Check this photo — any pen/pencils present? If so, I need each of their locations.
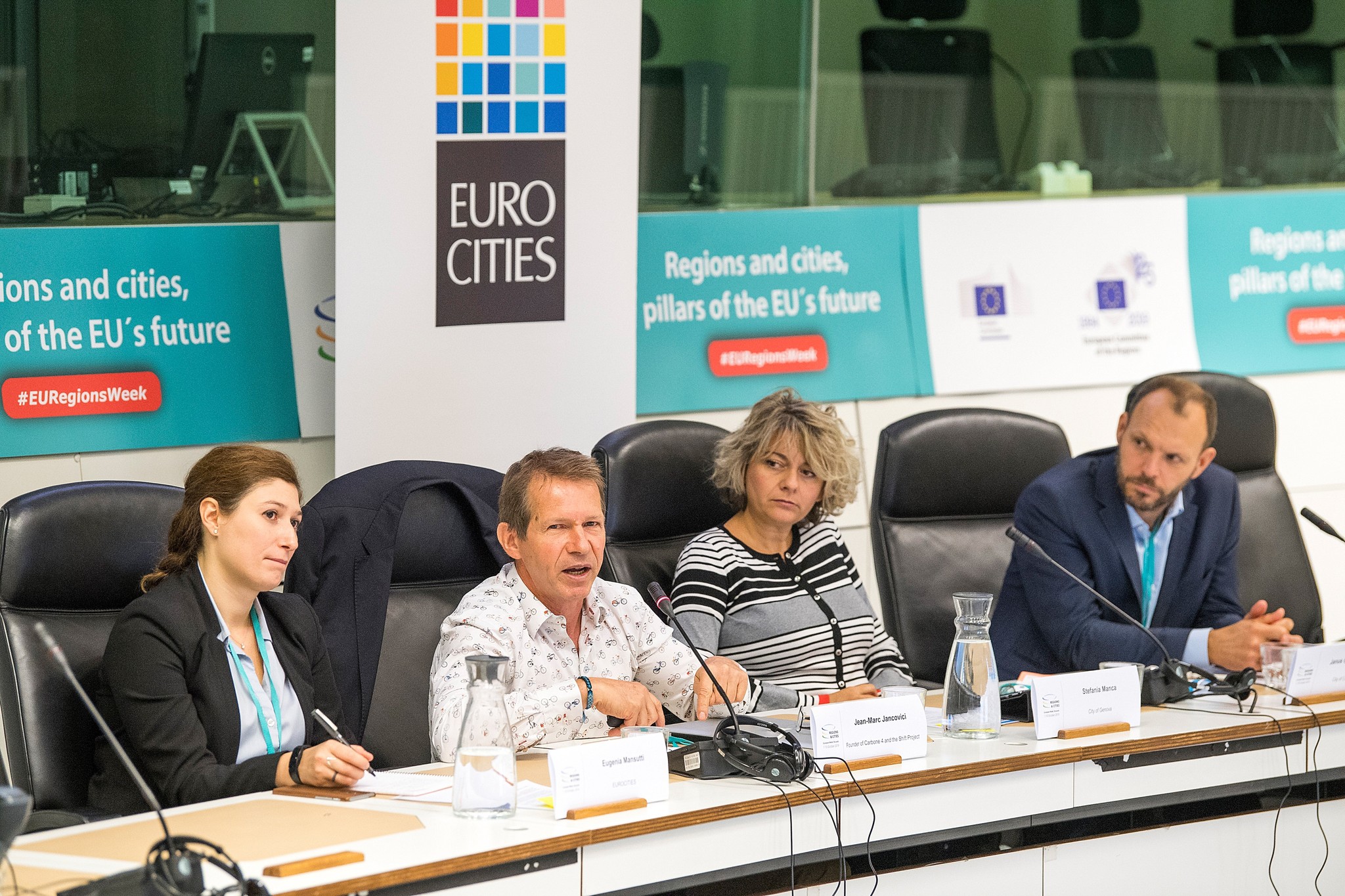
[311,708,377,778]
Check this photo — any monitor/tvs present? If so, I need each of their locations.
[181,28,316,176]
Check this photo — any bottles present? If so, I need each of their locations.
[941,592,1001,740]
[451,655,518,819]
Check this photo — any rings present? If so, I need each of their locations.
[332,771,339,782]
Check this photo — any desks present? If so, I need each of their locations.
[0,663,1345,896]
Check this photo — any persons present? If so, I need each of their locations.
[80,445,374,803]
[667,387,915,707]
[989,374,1308,682]
[425,447,751,766]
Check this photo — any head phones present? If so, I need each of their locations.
[143,834,272,895]
[712,715,816,784]
[1159,655,1257,702]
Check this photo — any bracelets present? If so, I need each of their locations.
[574,674,594,722]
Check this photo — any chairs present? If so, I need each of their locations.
[1214,0,1342,175]
[869,408,1073,689]
[1124,372,1323,645]
[859,0,1006,176]
[282,458,511,772]
[0,476,198,838]
[590,418,746,626]
[639,13,687,198]
[1071,1,1179,178]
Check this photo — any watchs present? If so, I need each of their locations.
[288,745,311,784]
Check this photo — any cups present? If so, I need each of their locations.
[1260,641,1303,690]
[621,725,670,751]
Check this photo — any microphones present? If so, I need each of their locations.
[953,28,1034,191]
[644,581,781,779]
[1001,527,1191,705]
[30,617,182,895]
[1298,508,1345,543]
[1193,35,1272,188]
